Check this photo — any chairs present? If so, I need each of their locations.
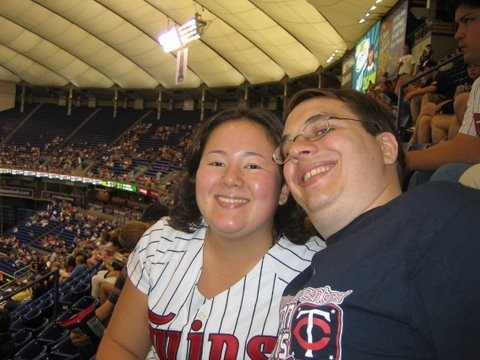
[0,44,480,359]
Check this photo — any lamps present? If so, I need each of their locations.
[156,15,204,54]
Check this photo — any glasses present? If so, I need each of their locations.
[273,118,370,166]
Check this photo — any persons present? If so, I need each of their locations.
[366,0,479,189]
[0,117,198,195]
[1,201,171,347]
[96,107,330,360]
[279,87,480,360]
